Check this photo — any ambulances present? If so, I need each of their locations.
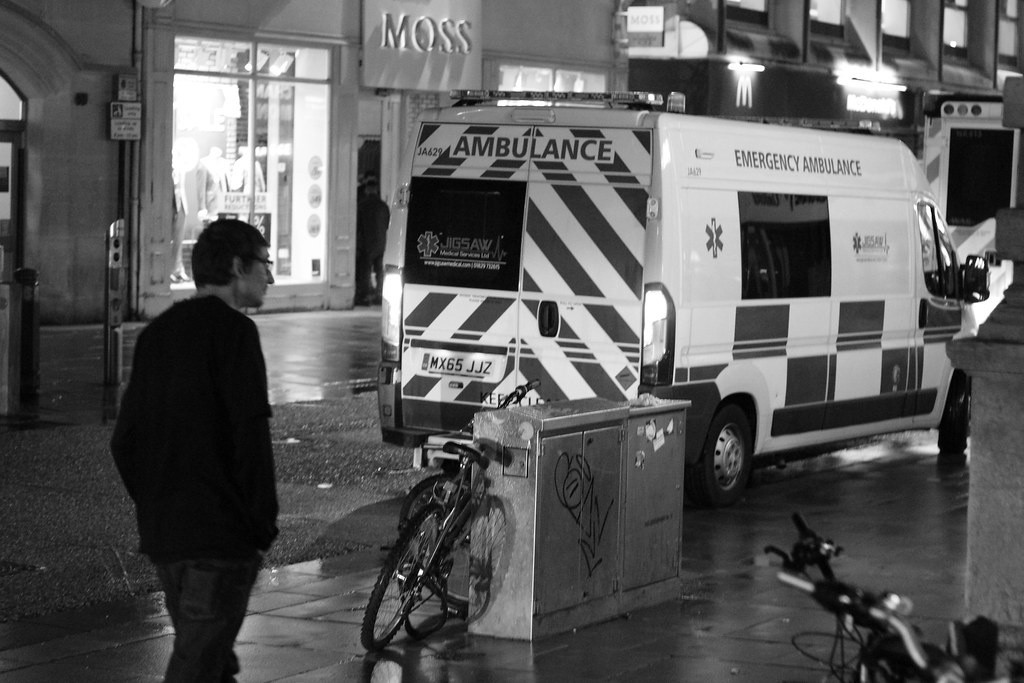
[385,105,990,508]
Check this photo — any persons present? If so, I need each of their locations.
[110,219,282,683]
[358,177,391,306]
[170,145,267,283]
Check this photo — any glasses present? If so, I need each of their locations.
[244,254,273,272]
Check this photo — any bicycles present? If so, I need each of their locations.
[360,377,542,654]
[763,511,1024,683]
